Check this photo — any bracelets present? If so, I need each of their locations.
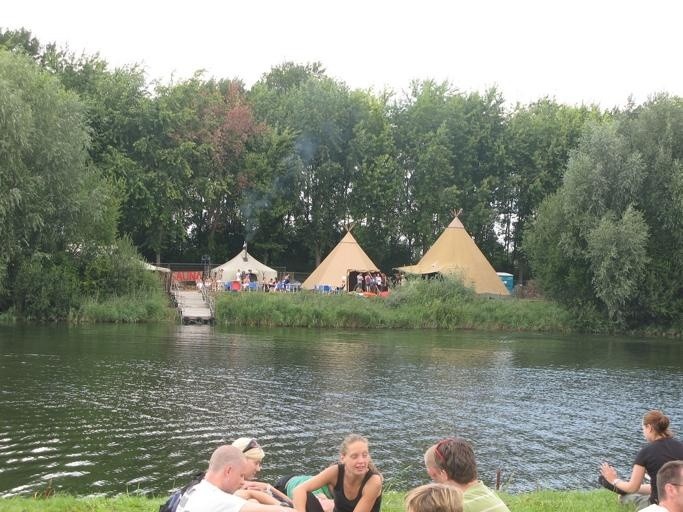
[612,478,620,486]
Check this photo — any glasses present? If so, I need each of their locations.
[435,439,452,463]
[242,440,258,454]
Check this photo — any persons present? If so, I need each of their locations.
[268,273,290,291]
[638,460,683,512]
[424,437,511,512]
[404,483,465,512]
[173,445,302,512]
[236,268,253,283]
[272,472,336,512]
[357,273,409,295]
[195,275,204,290]
[231,437,294,506]
[291,432,383,512]
[597,409,683,508]
